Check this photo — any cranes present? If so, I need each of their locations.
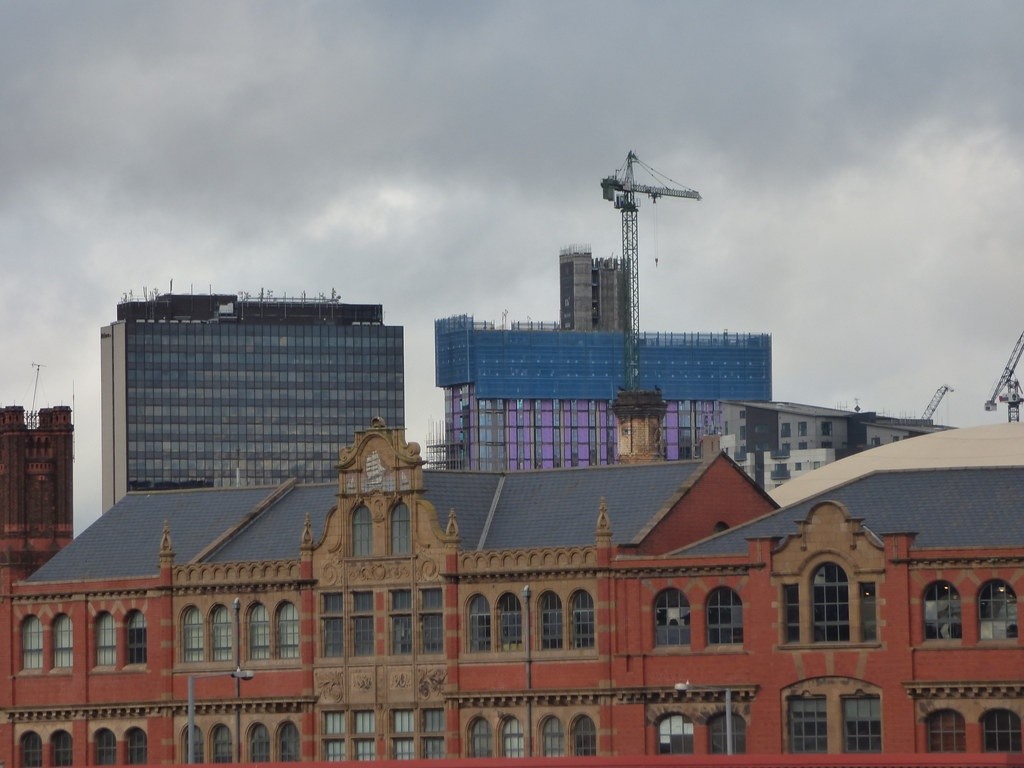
[921,385,957,425]
[986,330,1024,426]
[600,148,702,391]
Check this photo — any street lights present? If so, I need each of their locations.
[675,682,733,756]
[186,669,253,767]
